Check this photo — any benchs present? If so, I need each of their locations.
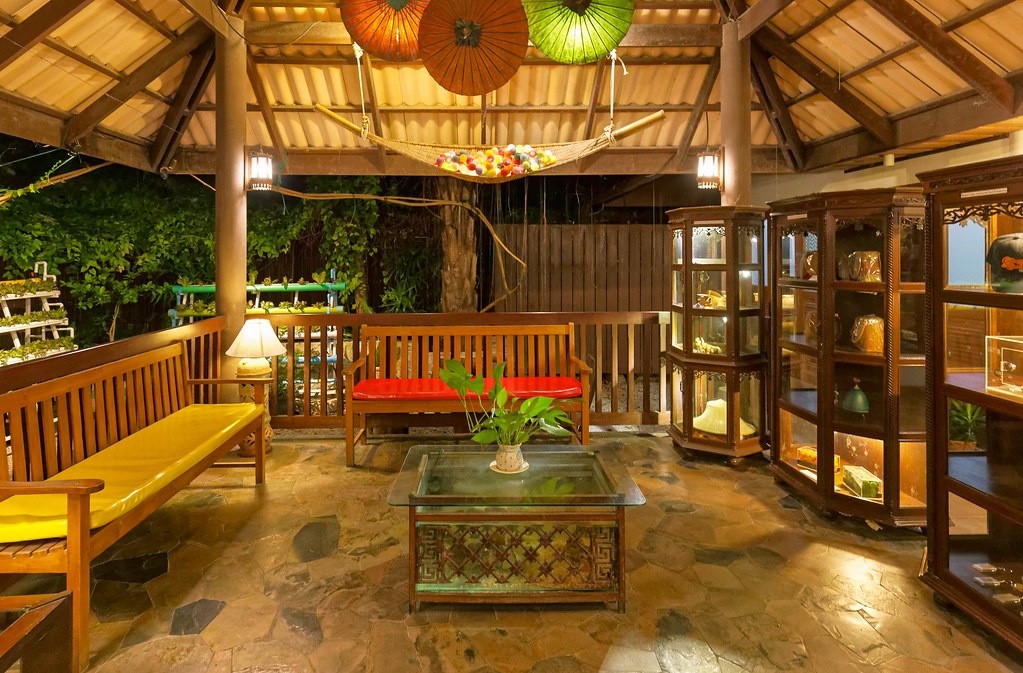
[0,338,276,673]
[341,322,593,465]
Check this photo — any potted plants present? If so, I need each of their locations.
[438,360,585,471]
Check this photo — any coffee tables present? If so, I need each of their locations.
[386,445,648,614]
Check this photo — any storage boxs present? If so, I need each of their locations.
[985,335,1023,398]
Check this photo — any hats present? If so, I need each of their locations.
[986,233,1023,293]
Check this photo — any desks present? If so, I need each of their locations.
[0,589,74,673]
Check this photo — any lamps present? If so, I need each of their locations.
[244,144,273,191]
[225,318,288,458]
[696,145,725,191]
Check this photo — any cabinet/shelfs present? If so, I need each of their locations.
[914,153,1023,652]
[766,185,955,529]
[665,205,770,468]
